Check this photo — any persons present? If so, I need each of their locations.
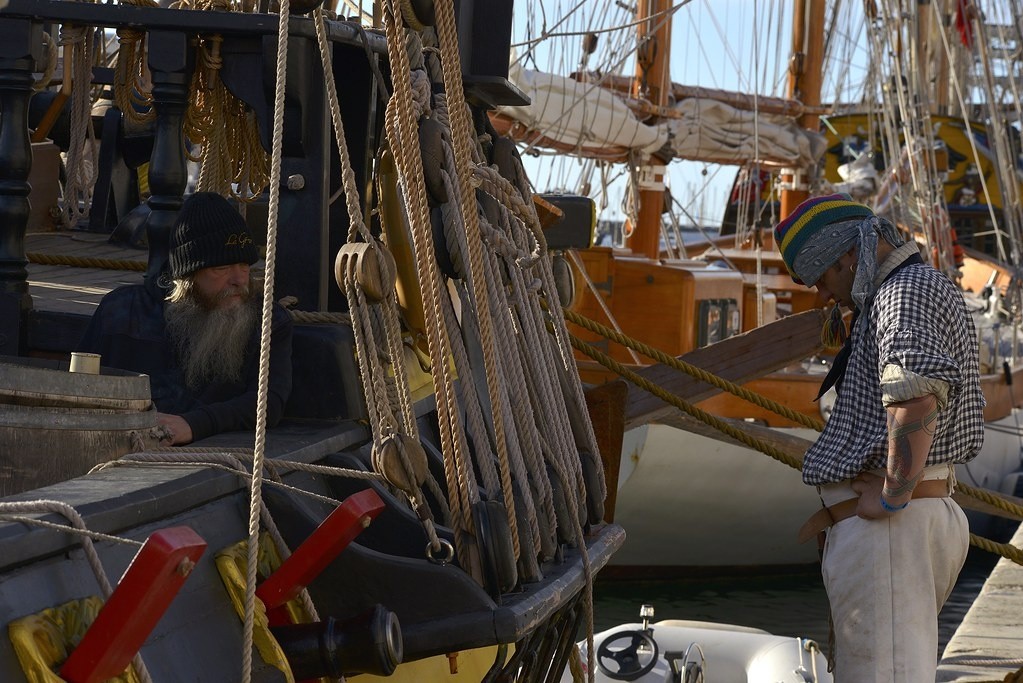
[773,194,985,683]
[77,194,293,446]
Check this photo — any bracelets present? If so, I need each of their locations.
[880,495,911,512]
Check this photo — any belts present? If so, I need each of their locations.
[798,479,949,545]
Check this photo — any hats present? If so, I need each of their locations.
[169,192,260,278]
[774,193,875,282]
